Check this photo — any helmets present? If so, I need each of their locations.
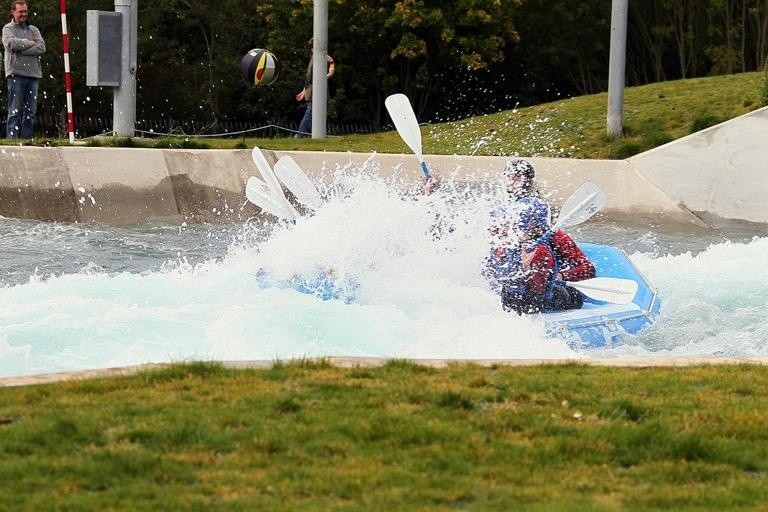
[504,158,536,179]
[510,196,550,228]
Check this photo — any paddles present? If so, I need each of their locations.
[555,278,638,305]
[385,94,437,193]
[245,146,322,225]
[496,181,606,281]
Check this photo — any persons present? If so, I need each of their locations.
[2,0,46,139]
[481,159,596,316]
[418,174,435,196]
[294,37,335,138]
